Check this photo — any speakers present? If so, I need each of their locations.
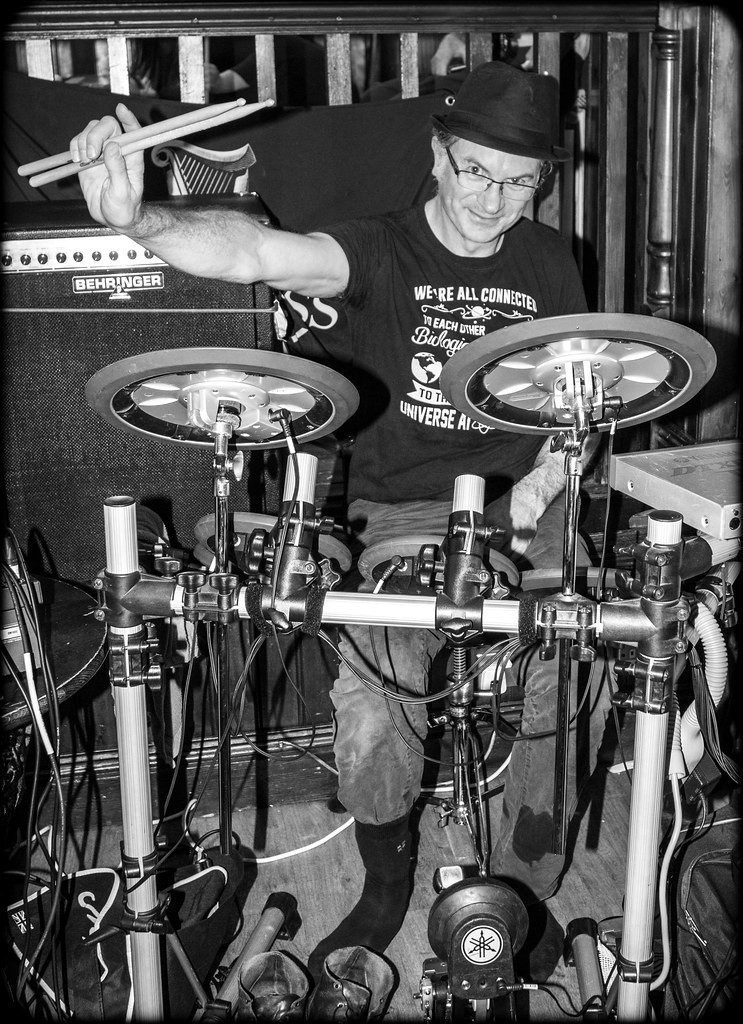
[0,192,302,747]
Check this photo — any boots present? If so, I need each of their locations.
[236,945,396,1024]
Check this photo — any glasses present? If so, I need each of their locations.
[445,144,541,201]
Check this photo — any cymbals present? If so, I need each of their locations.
[435,305,721,440]
[79,341,369,456]
[355,532,523,605]
[520,559,646,605]
[191,506,358,584]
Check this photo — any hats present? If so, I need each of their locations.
[431,60,573,165]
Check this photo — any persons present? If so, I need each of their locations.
[69,60,618,990]
